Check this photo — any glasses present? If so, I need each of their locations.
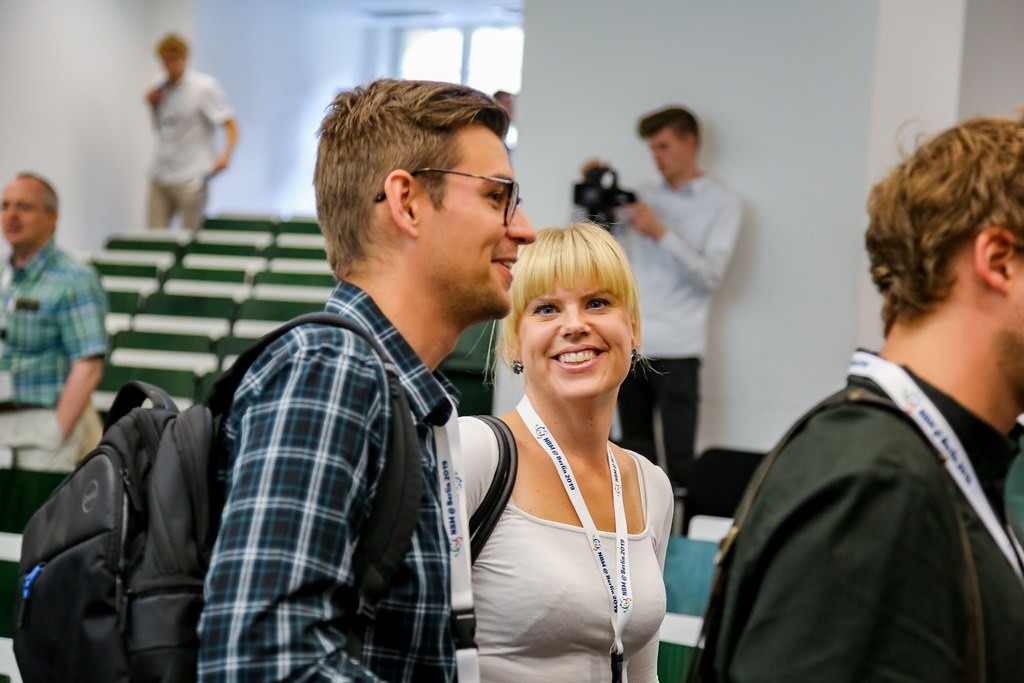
[374,167,521,227]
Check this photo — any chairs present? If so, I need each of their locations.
[1,215,767,683]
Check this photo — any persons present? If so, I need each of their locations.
[198,78,536,683]
[1,172,107,469]
[457,223,674,683]
[583,108,742,482]
[148,34,237,231]
[690,116,1024,683]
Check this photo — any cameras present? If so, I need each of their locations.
[574,167,637,211]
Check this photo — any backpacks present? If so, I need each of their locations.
[13,309,424,683]
